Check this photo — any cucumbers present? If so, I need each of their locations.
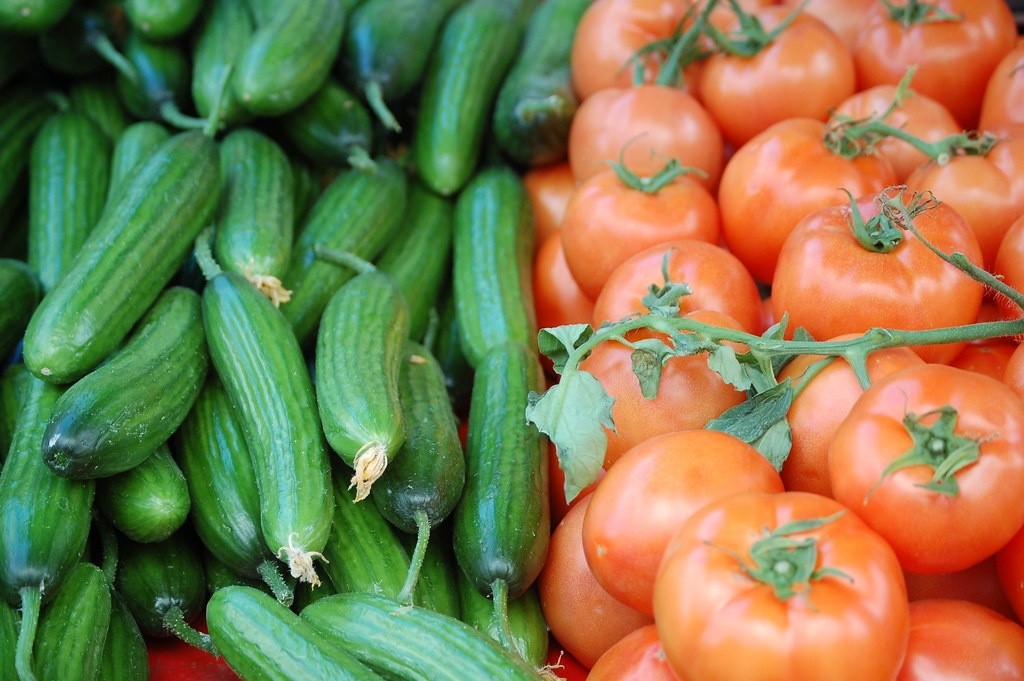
[0,0,595,681]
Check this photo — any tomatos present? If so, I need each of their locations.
[536,0,1024,680]
